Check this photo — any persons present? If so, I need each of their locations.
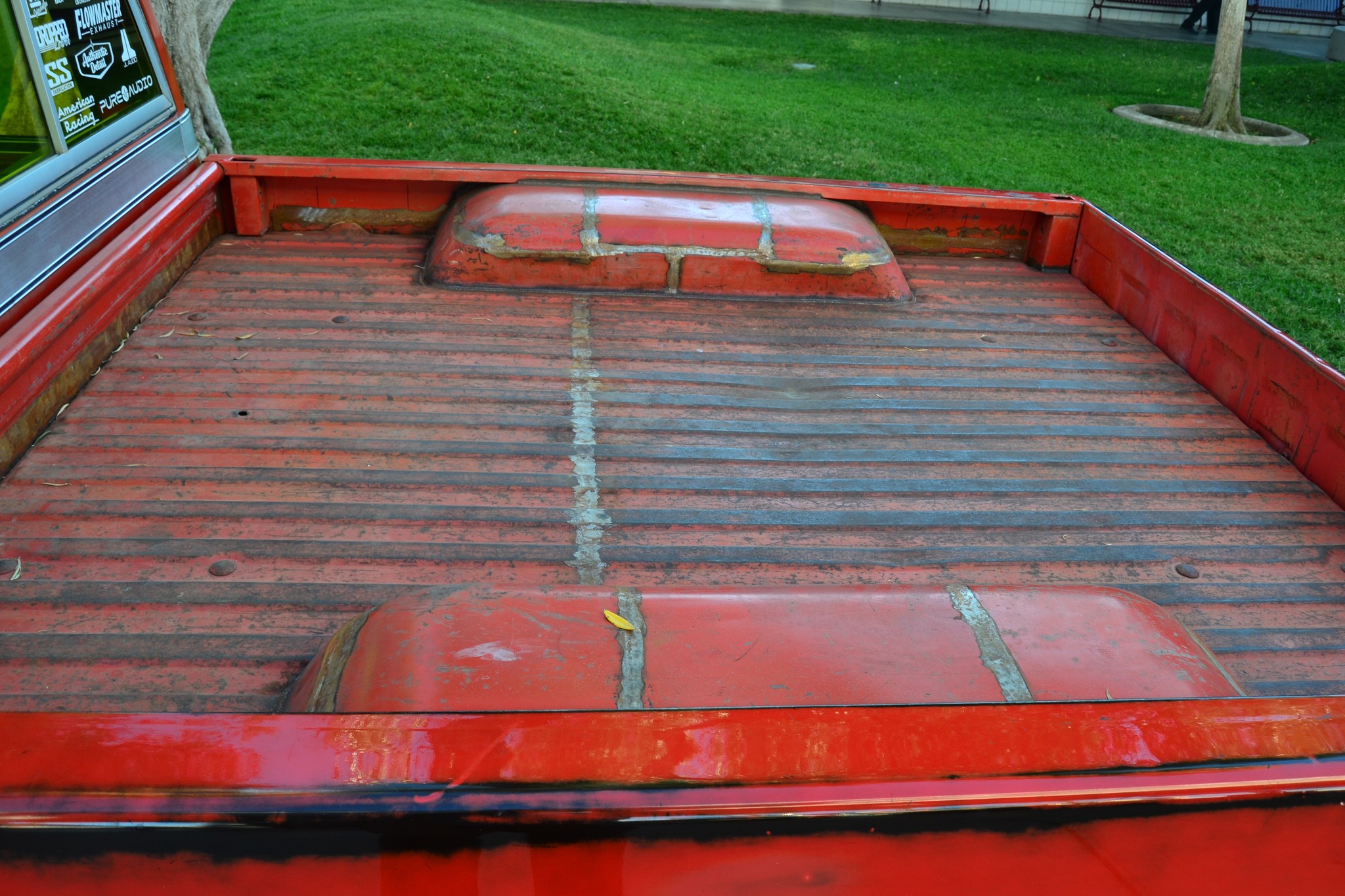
[1181,0,1221,37]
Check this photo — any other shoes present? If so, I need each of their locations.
[1206,30,1219,35]
[1180,19,1198,34]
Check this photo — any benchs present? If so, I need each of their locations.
[1087,0,1203,31]
[1245,0,1345,38]
